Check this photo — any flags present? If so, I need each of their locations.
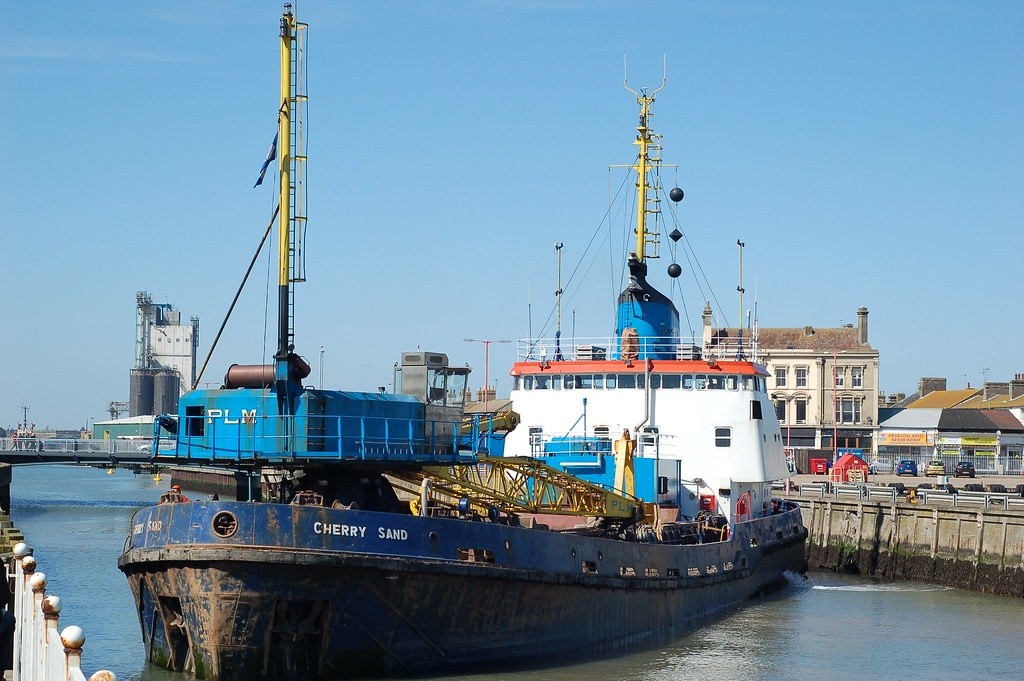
[253,127,278,189]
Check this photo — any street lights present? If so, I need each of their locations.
[319,345,325,391]
[463,338,513,414]
[922,430,927,465]
[86,416,95,433]
[784,395,794,452]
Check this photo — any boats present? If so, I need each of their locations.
[118,54,809,681]
[10,405,38,452]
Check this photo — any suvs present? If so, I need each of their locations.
[953,461,975,478]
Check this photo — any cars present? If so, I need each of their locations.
[924,460,946,477]
[868,459,897,475]
[896,460,917,476]
[137,439,175,454]
